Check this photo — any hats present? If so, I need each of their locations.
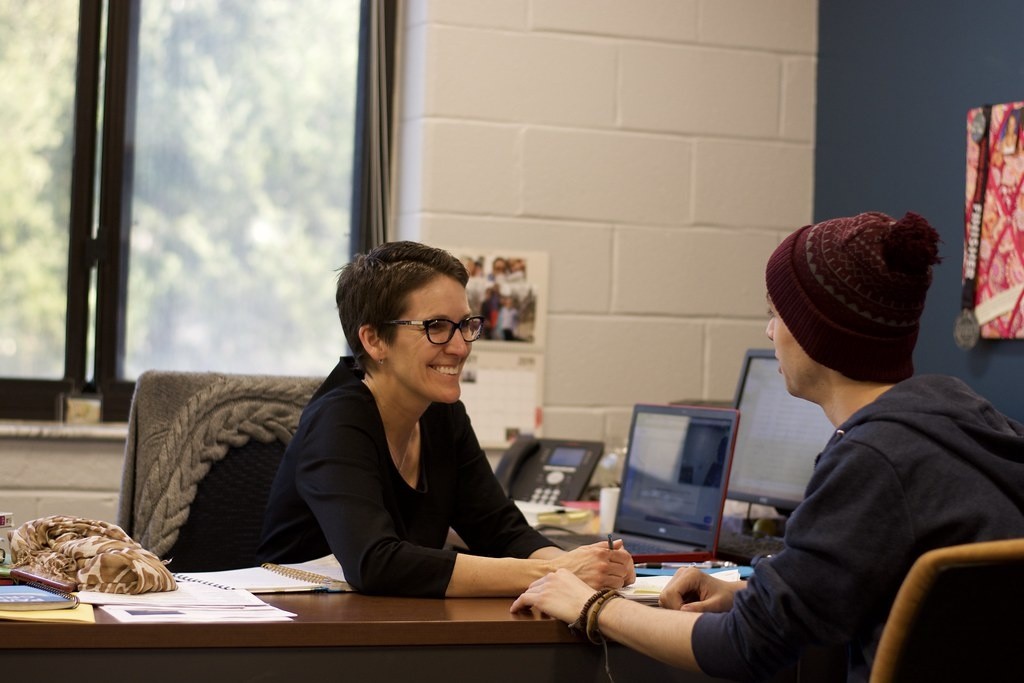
[766,210,946,386]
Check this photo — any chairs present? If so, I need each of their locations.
[115,368,326,573]
[870,538,1024,683]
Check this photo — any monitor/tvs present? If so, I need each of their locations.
[724,348,838,511]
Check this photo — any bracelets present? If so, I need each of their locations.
[568,588,625,645]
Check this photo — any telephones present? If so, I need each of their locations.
[494,435,604,507]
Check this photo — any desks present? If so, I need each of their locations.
[0,587,692,683]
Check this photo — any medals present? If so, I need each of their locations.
[955,311,980,349]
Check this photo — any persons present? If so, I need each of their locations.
[258,241,636,600]
[1001,115,1017,155]
[509,212,1024,683]
[459,254,532,342]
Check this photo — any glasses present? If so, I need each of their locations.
[382,315,485,345]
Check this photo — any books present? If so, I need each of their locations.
[0,581,80,611]
[173,554,358,593]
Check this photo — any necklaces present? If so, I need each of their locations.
[399,426,415,473]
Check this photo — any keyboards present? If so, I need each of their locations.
[716,527,788,564]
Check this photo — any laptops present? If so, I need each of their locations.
[543,403,741,564]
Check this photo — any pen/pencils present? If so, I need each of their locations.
[634,562,722,569]
[608,534,614,550]
